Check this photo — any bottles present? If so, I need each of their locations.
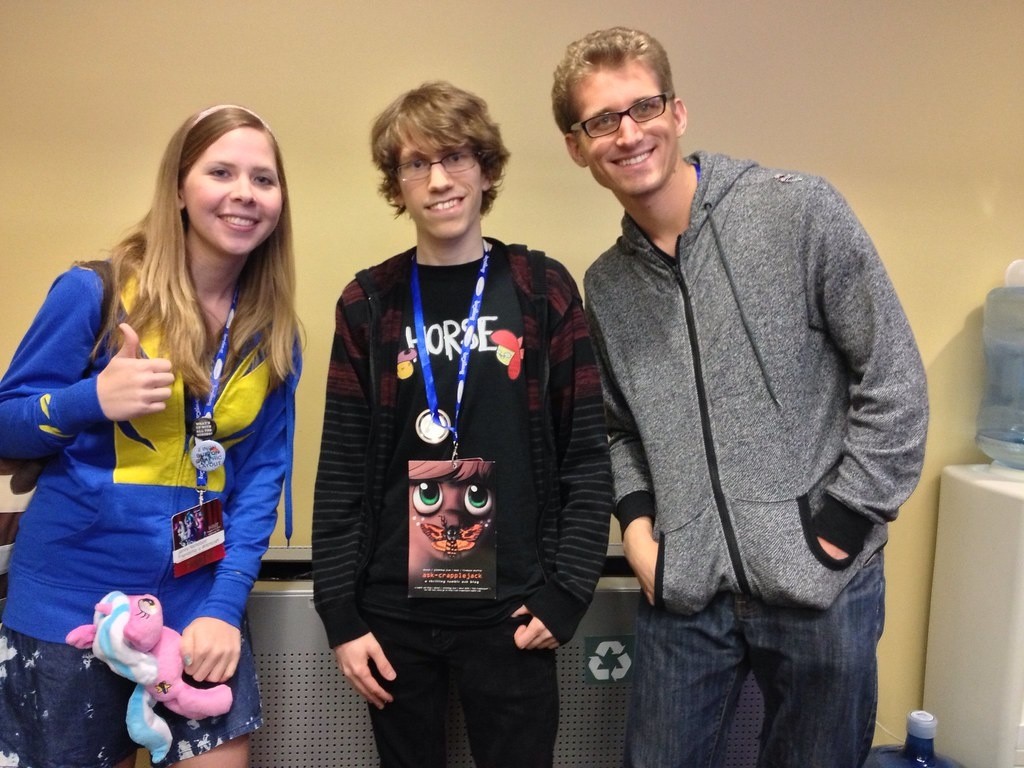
[976,287,1024,470]
[862,710,967,768]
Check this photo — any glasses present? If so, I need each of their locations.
[571,92,670,139]
[388,153,477,181]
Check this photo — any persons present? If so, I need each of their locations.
[306,78,615,768]
[0,102,297,768]
[551,22,931,768]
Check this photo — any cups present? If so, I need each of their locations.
[1005,260,1024,287]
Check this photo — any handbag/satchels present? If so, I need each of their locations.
[0,261,112,599]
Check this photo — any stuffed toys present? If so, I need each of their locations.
[65,590,234,766]
